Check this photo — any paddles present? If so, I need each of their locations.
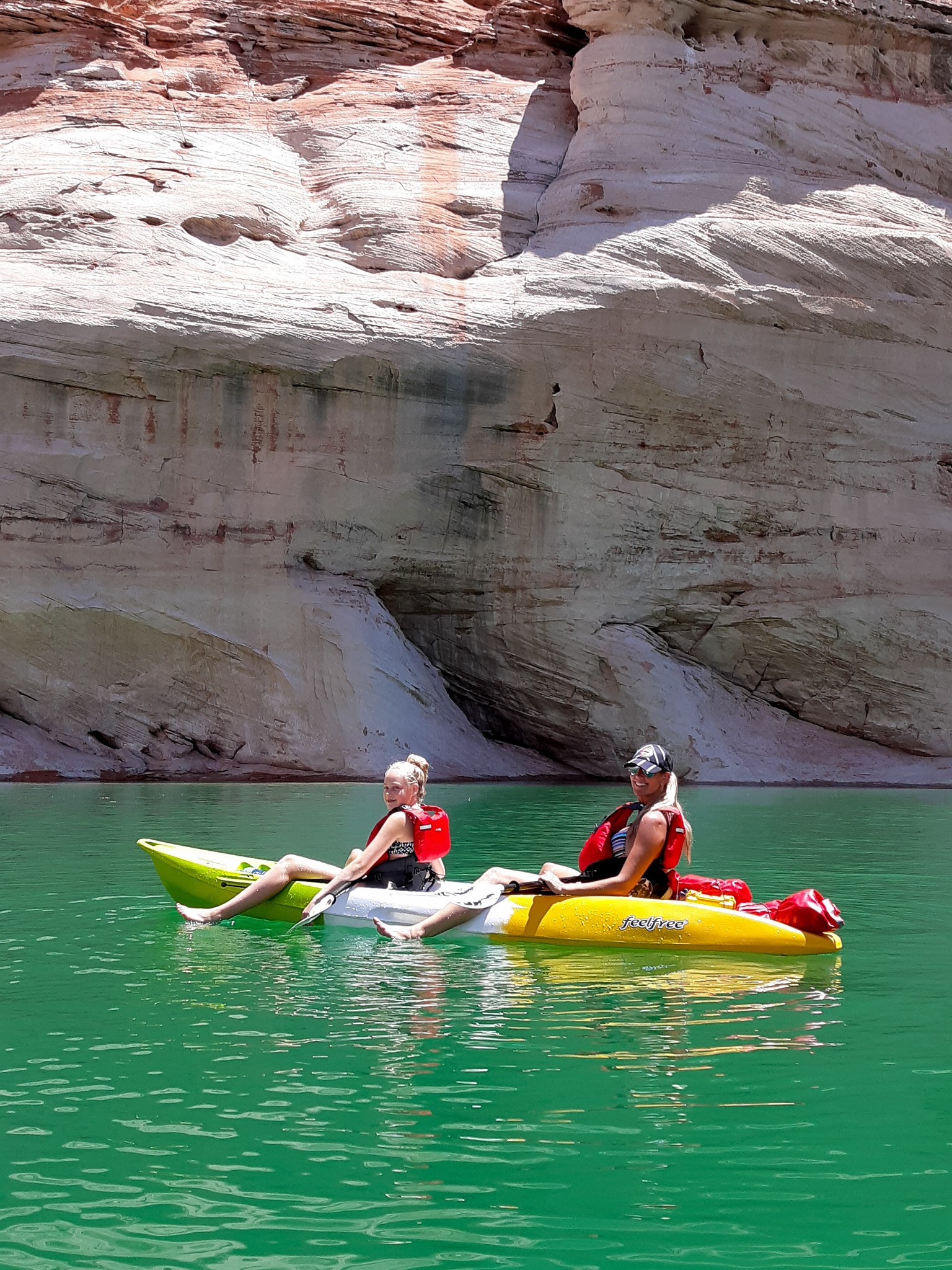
[287,877,363,936]
[443,872,586,911]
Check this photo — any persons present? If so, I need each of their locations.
[176,754,446,926]
[373,745,692,939]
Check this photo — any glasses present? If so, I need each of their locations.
[628,764,666,778]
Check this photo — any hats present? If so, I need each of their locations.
[622,743,675,774]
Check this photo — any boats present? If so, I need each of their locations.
[134,836,846,958]
